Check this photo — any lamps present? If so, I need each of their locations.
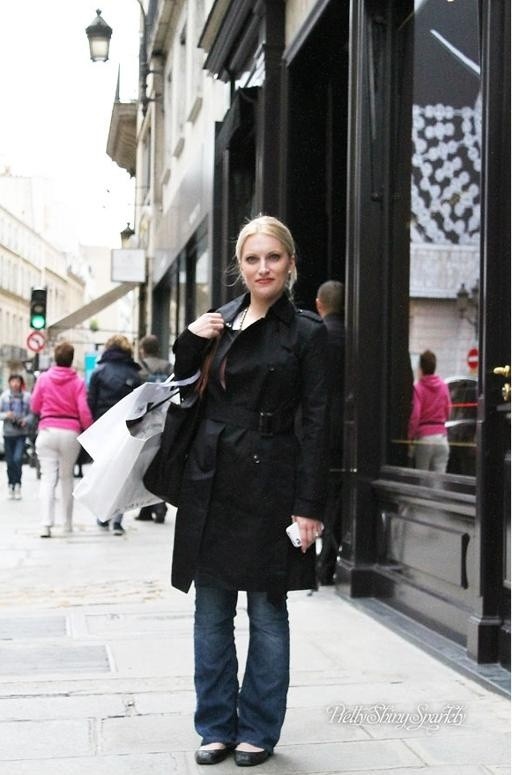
[120,222,134,249]
[85,1,165,116]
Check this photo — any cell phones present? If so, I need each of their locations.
[286,521,324,548]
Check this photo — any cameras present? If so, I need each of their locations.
[10,416,22,429]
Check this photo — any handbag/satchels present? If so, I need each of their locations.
[74,378,198,526]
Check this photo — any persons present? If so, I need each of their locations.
[170,213,334,767]
[314,280,345,588]
[406,349,452,490]
[0,334,174,538]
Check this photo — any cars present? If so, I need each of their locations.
[437,376,478,474]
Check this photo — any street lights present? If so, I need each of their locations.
[457,277,479,342]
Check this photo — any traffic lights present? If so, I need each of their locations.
[28,288,48,331]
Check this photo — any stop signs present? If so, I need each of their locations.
[467,348,479,369]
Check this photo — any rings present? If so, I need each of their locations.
[314,531,321,537]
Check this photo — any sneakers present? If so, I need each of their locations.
[62,522,74,532]
[97,519,124,535]
[39,525,52,538]
[8,481,23,499]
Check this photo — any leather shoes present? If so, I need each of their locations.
[231,743,272,767]
[195,739,236,765]
